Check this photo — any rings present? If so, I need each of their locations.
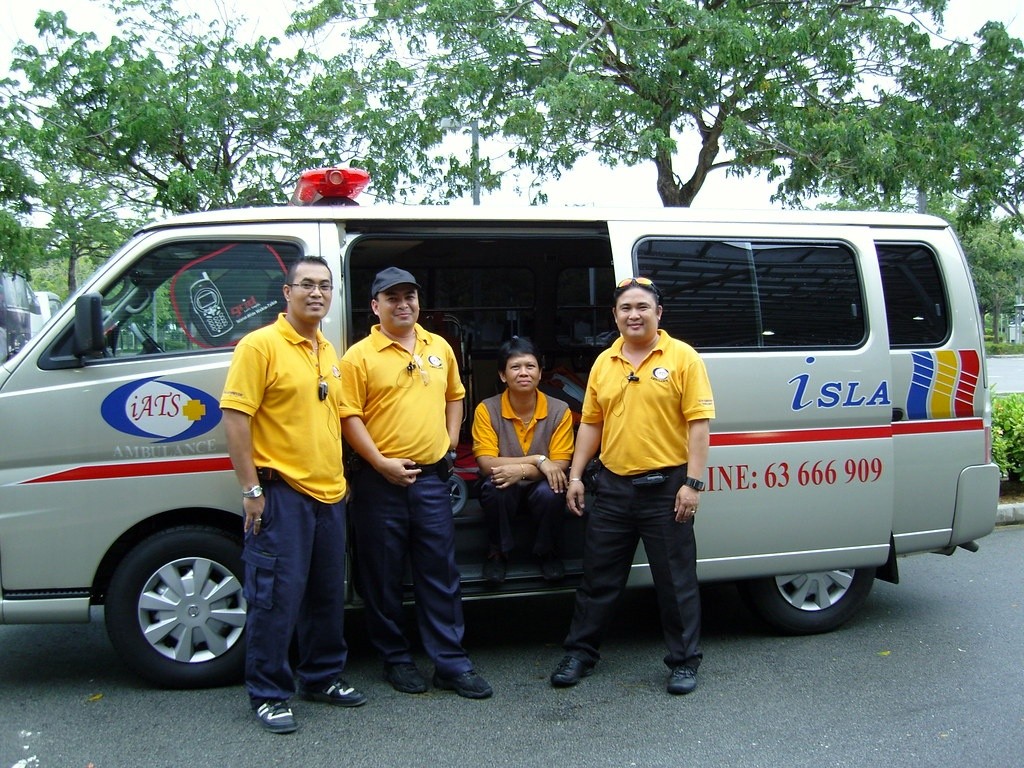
[696,509,698,511]
[691,511,695,514]
[255,518,261,522]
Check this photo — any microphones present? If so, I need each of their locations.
[409,361,413,373]
[629,371,634,383]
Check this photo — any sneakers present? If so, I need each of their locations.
[550,654,593,685]
[382,661,428,693]
[298,675,368,707]
[539,556,566,581]
[432,667,492,699]
[667,664,698,695]
[256,698,298,733]
[481,546,507,582]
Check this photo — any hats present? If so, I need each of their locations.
[372,267,422,299]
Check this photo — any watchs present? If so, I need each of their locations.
[537,455,546,468]
[447,447,458,459]
[684,476,705,491]
[242,485,263,498]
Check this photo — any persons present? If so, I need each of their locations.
[472,337,575,582]
[219,257,368,733]
[551,277,716,693]
[339,267,493,699]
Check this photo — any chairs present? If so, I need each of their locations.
[261,275,288,325]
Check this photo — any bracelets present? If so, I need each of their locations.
[519,463,526,480]
[568,478,580,484]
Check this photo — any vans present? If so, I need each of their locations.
[0,168,1000,690]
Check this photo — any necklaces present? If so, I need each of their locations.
[314,348,319,350]
[512,403,536,424]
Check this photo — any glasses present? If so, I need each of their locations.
[287,281,334,292]
[614,277,660,301]
